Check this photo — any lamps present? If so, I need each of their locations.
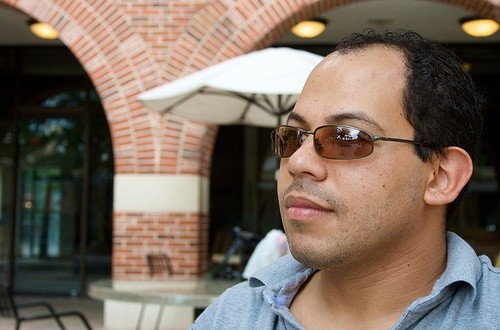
[457,14,500,38]
[290,16,328,39]
[25,18,59,40]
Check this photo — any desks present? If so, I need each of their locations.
[88,275,243,330]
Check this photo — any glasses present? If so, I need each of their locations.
[269,125,443,161]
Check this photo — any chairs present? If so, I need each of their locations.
[0,261,93,330]
[212,239,242,278]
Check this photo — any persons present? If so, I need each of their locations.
[186,26,500,330]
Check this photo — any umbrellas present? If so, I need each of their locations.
[136,45,328,173]
